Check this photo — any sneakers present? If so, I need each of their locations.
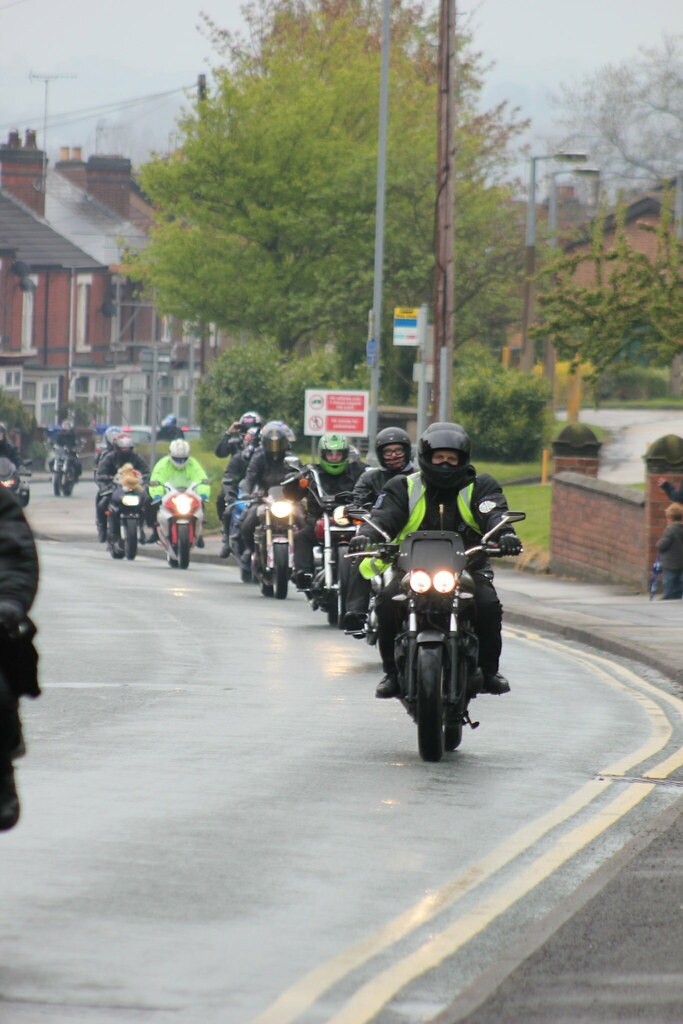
[484,672,511,695]
[375,671,400,699]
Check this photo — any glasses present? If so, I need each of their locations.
[383,448,406,456]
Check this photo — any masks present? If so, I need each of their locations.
[383,456,406,469]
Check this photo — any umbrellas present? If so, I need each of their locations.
[649,552,661,602]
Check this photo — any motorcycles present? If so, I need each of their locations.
[147,478,212,568]
[0,456,33,509]
[346,509,527,761]
[230,491,302,599]
[97,472,150,560]
[337,491,396,645]
[50,443,82,497]
[281,457,372,626]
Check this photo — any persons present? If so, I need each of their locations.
[0,430,41,829]
[216,412,521,700]
[654,476,683,601]
[44,414,212,572]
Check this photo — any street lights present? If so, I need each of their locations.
[519,152,590,365]
[545,167,602,376]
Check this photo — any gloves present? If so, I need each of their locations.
[498,533,522,557]
[349,535,371,557]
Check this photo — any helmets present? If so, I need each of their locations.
[0,423,7,442]
[374,426,411,471]
[61,421,74,436]
[161,415,177,428]
[240,411,295,461]
[105,426,122,446]
[169,439,190,469]
[319,433,349,475]
[113,434,134,455]
[418,422,476,490]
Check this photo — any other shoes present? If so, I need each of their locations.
[291,569,308,590]
[341,610,367,639]
[219,543,230,558]
[147,527,159,543]
[97,526,107,543]
[196,535,205,549]
[0,762,20,832]
[241,550,251,562]
